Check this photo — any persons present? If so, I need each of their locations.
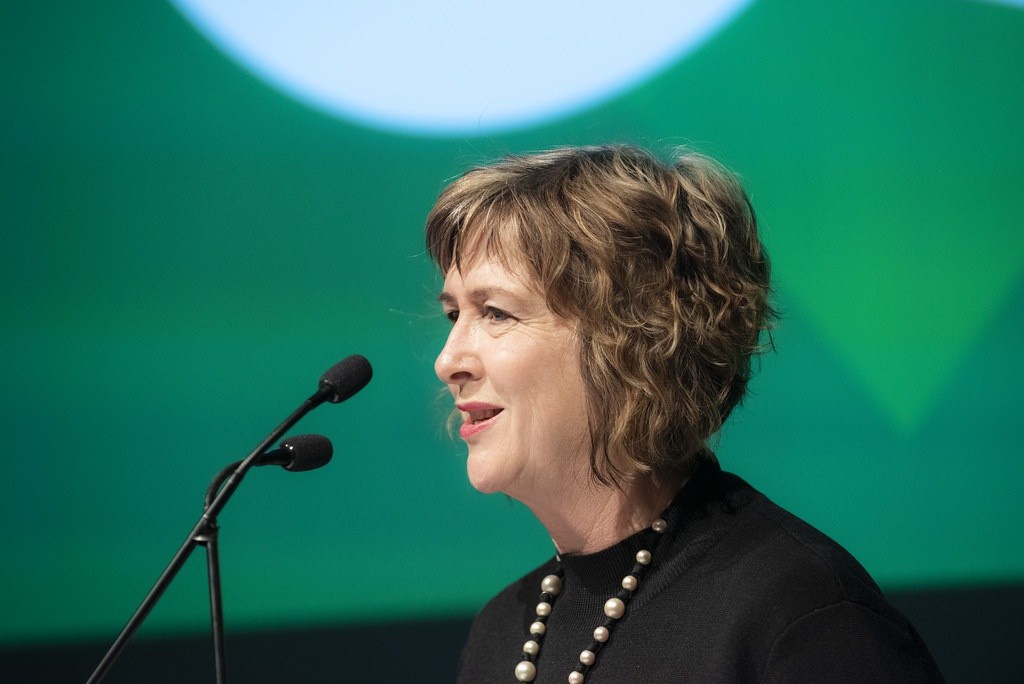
[420,146,952,684]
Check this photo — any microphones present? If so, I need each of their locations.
[85,354,375,684]
[201,432,333,683]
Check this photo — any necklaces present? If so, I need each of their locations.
[516,459,698,683]
